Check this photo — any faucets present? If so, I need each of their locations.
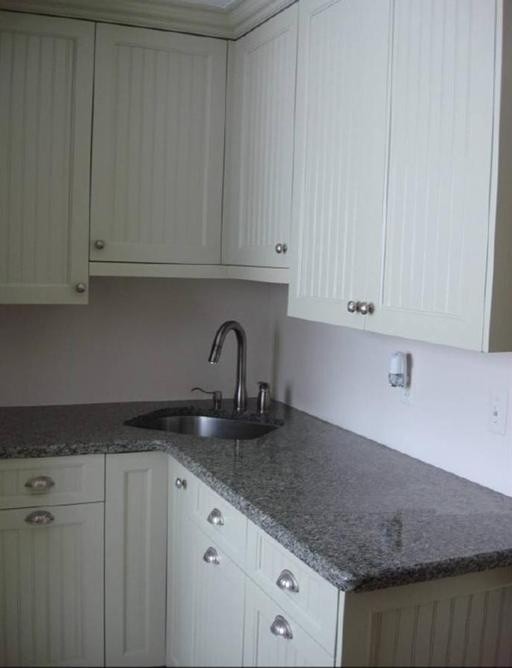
[209,322,248,410]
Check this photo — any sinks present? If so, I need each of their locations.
[122,410,285,443]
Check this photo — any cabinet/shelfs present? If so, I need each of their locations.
[105,450,166,667]
[220,1,299,288]
[166,454,511,668]
[0,454,104,668]
[89,22,220,278]
[0,10,96,304]
[285,0,511,354]
